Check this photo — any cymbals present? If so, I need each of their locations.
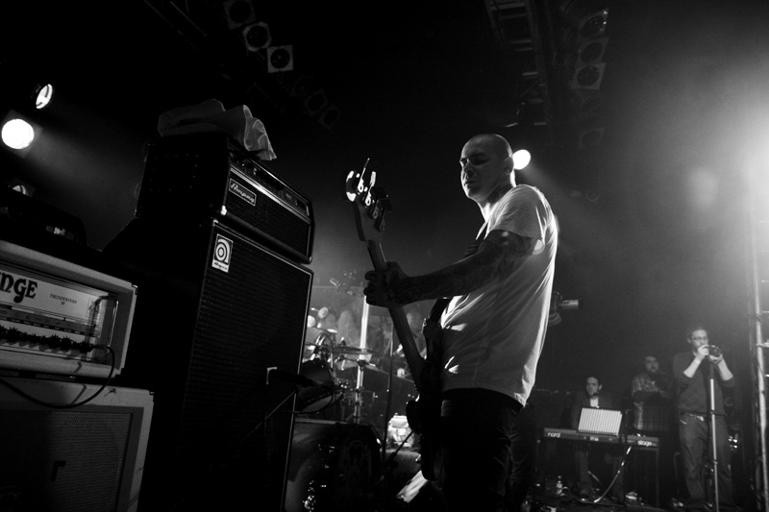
[307,344,376,355]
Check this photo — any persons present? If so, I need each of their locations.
[672,325,736,511]
[362,133,560,512]
[567,376,619,503]
[626,355,675,500]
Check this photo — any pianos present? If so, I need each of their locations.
[545,428,660,450]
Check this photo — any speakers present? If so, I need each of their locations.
[105,219,313,512]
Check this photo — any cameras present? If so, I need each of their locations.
[706,344,723,357]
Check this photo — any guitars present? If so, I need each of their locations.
[345,157,443,481]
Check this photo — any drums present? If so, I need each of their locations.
[297,361,343,416]
